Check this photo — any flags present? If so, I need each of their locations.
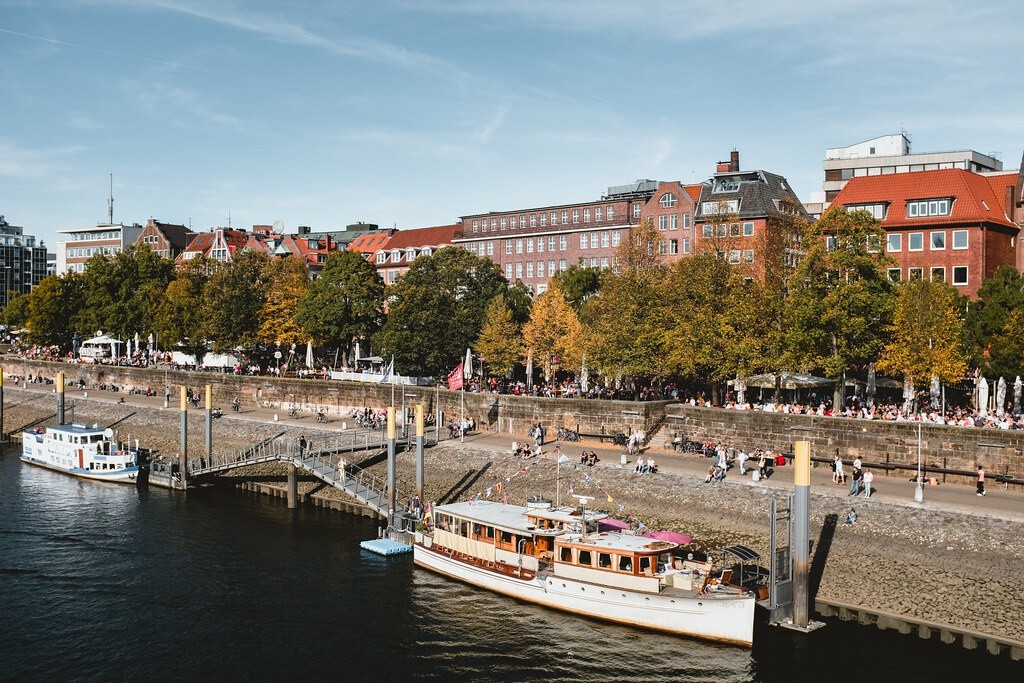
[424,504,431,526]
[448,363,462,390]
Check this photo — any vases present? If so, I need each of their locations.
[694,574,699,578]
[744,592,748,595]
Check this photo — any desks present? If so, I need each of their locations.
[764,457,778,466]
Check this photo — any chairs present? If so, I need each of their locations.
[715,569,733,585]
[644,567,665,583]
[694,575,710,596]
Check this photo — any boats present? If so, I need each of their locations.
[17,421,140,484]
[412,443,756,649]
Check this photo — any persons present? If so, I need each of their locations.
[0,341,1024,524]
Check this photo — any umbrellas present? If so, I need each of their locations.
[929,372,940,407]
[148,333,153,356]
[1013,375,1021,413]
[581,353,588,392]
[526,346,533,390]
[544,350,552,386]
[604,376,612,388]
[110,340,118,362]
[997,376,1006,416]
[598,518,692,545]
[134,332,140,357]
[979,377,989,418]
[866,361,876,401]
[903,373,915,418]
[126,338,132,364]
[305,342,314,369]
[614,372,623,390]
[463,347,474,384]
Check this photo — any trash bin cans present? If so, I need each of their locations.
[778,456,785,467]
[729,448,734,458]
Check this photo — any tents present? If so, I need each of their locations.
[727,370,839,404]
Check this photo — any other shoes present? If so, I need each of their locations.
[977,493,982,496]
[982,491,986,496]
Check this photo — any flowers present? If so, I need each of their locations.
[693,570,698,574]
[742,586,748,592]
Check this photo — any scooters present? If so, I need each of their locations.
[314,409,328,424]
[287,408,299,419]
